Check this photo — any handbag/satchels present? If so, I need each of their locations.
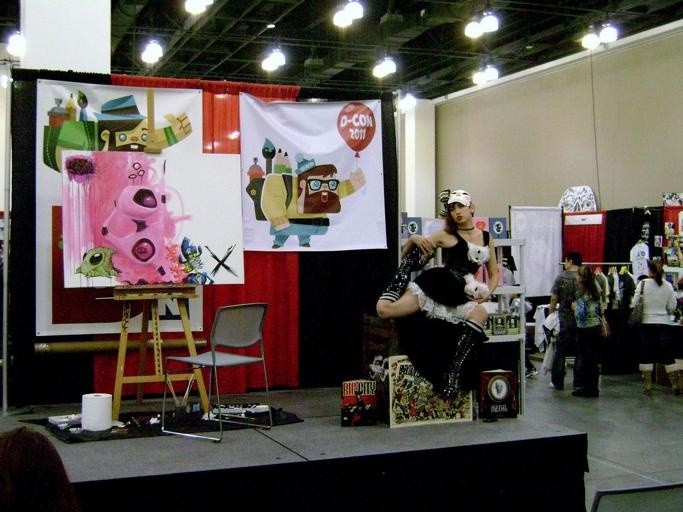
[600,314,609,337]
[628,303,642,325]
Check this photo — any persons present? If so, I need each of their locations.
[509,284,538,378]
[549,253,583,390]
[572,266,603,397]
[376,191,499,399]
[630,260,680,395]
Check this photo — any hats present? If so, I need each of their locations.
[448,190,472,206]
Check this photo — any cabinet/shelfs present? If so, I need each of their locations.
[476,237,529,415]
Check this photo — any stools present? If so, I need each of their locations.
[111,282,209,422]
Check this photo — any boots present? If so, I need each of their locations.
[380,242,434,302]
[444,319,481,398]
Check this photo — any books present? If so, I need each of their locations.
[342,379,379,427]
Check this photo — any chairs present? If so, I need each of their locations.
[158,300,273,444]
[0,425,74,511]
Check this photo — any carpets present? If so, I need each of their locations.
[14,401,305,445]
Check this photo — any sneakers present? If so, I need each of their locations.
[525,369,538,377]
[548,382,599,398]
